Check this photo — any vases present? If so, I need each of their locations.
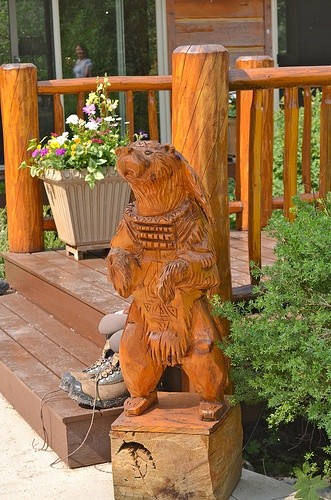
[33,166,132,261]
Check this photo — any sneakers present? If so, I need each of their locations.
[59,331,129,408]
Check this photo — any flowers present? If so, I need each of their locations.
[18,70,148,189]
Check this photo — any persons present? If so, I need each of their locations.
[72,44,93,78]
